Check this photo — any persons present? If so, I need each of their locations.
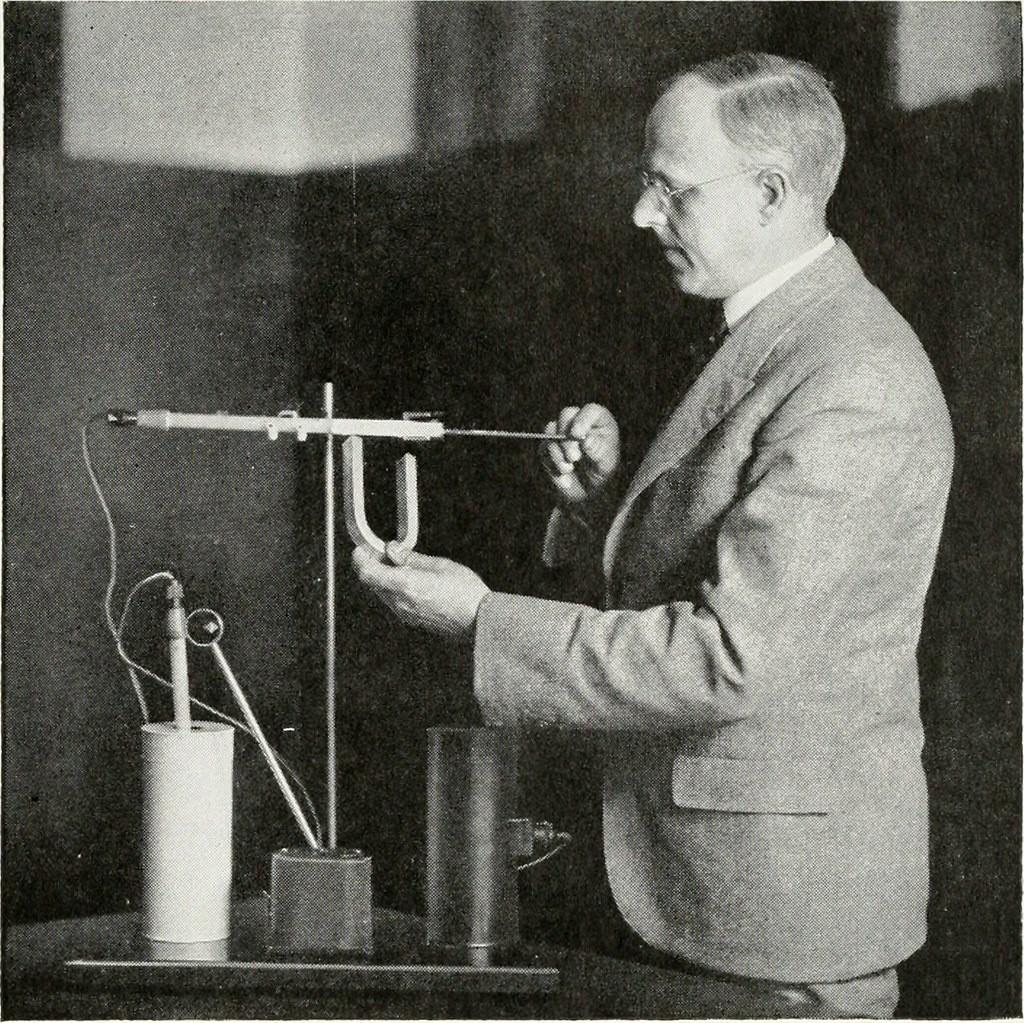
[348,50,957,1021]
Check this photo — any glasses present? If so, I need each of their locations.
[634,163,778,212]
